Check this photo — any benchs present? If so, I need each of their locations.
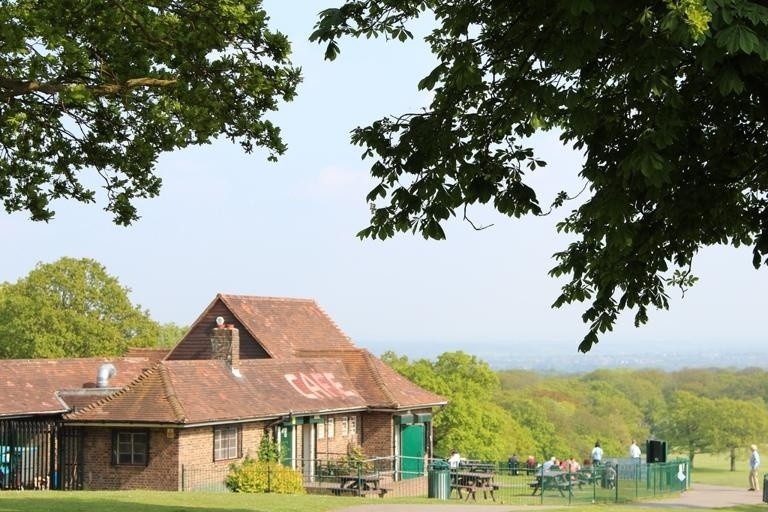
[373,487,393,498]
[450,471,602,502]
[326,488,359,496]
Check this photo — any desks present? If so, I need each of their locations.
[461,463,496,473]
[337,476,384,498]
[576,468,606,485]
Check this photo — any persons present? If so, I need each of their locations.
[525,455,535,475]
[447,448,461,480]
[629,440,641,462]
[507,454,519,475]
[538,456,595,485]
[604,461,617,491]
[591,442,602,466]
[747,444,759,491]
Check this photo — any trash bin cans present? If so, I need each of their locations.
[763,474,768,502]
[428,460,451,498]
